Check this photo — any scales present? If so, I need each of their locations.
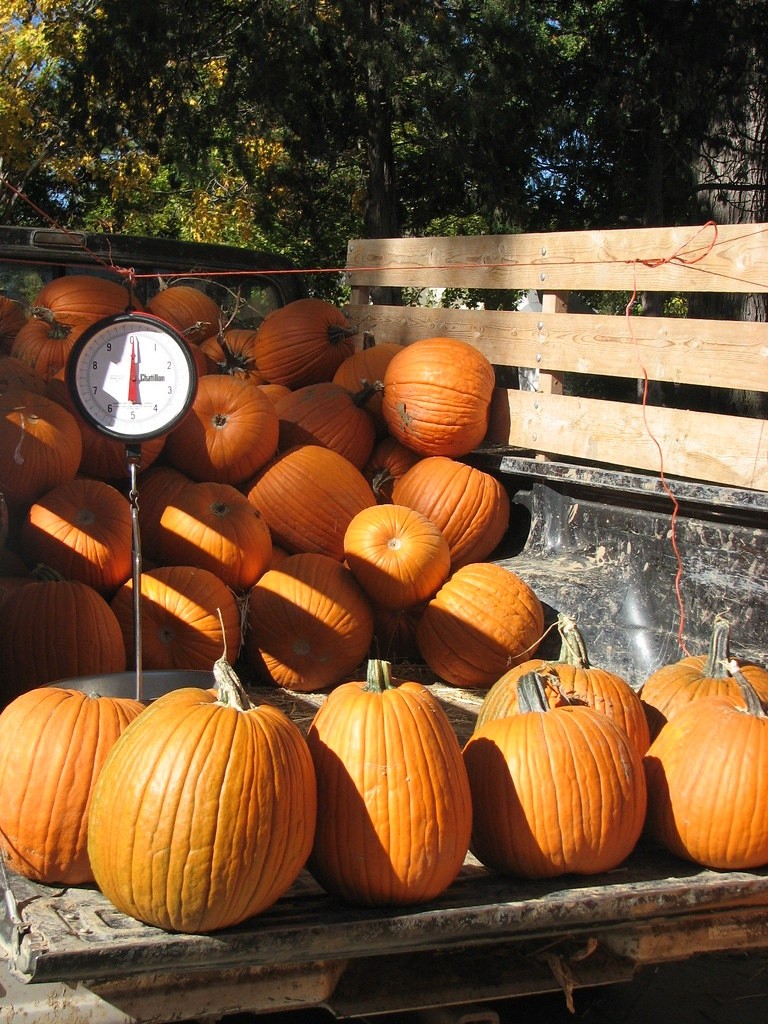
[37,309,220,706]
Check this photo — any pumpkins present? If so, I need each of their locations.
[460,671,648,883]
[633,620,768,748]
[304,659,471,910]
[0,687,150,886]
[88,661,317,933]
[0,276,544,691]
[643,660,768,871]
[477,613,650,761]
